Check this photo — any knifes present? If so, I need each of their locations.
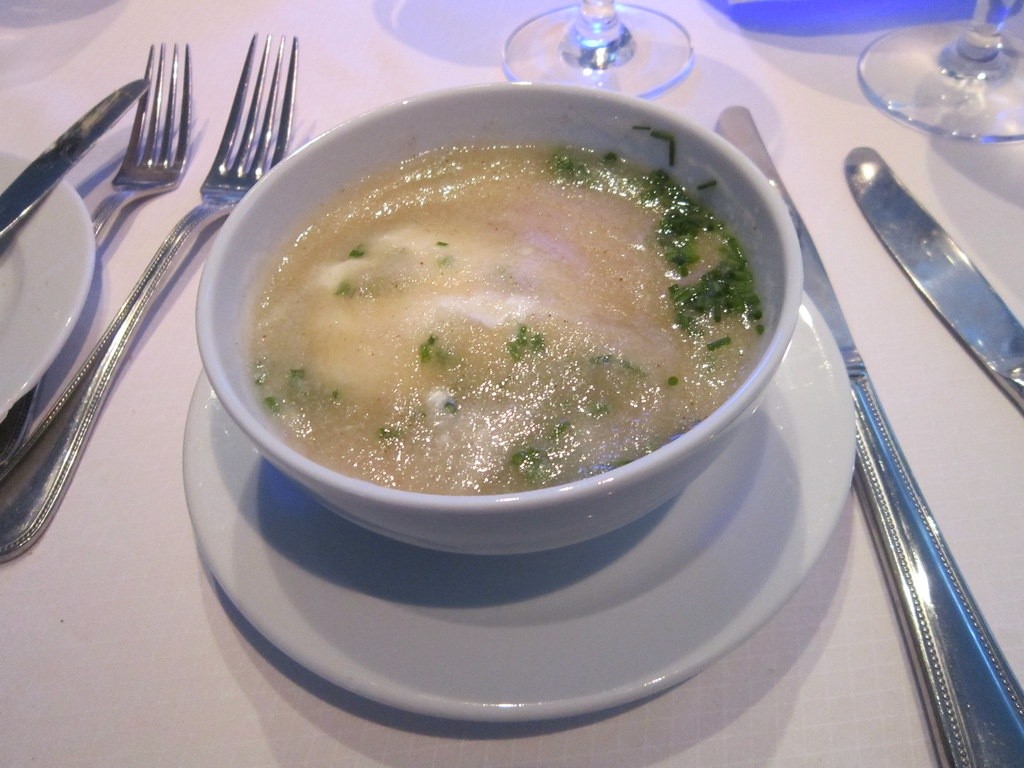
[0,75,152,264]
[714,105,1024,768]
[844,146,1024,426]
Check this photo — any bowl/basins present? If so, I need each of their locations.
[194,80,804,556]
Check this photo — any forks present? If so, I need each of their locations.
[0,33,300,562]
[0,41,191,568]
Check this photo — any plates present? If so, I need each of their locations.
[186,283,861,721]
[0,147,96,424]
